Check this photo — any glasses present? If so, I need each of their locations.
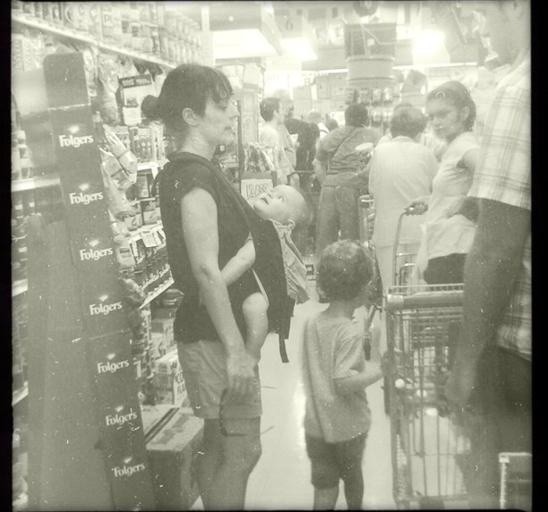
[427,109,457,121]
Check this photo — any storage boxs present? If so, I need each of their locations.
[138,404,204,511]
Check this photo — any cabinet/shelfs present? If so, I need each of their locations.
[214,57,265,202]
[12,0,200,512]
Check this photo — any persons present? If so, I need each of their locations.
[313,101,381,263]
[194,184,309,373]
[366,105,440,299]
[324,114,340,132]
[367,105,441,163]
[139,60,264,512]
[280,95,320,183]
[258,93,300,196]
[309,106,330,150]
[299,238,386,511]
[402,78,488,292]
[443,1,530,512]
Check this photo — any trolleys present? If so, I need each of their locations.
[392,205,460,348]
[358,194,375,243]
[385,282,471,511]
